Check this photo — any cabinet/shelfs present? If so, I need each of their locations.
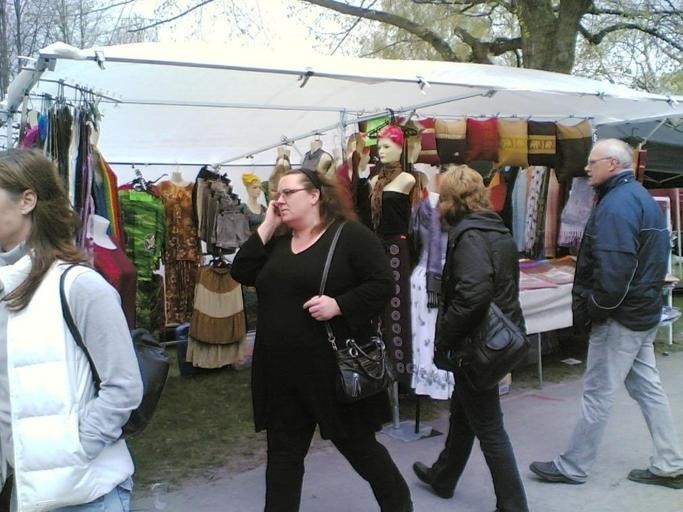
[653,196,682,346]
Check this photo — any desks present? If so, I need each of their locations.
[517,257,576,391]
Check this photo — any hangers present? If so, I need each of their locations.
[203,164,244,269]
[365,108,417,139]
[27,79,105,127]
[126,167,167,193]
[621,127,656,149]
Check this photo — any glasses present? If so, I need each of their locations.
[272,189,309,196]
[588,153,613,167]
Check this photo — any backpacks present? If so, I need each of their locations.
[333,336,398,402]
[60,264,170,433]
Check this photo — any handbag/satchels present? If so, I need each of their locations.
[450,303,532,391]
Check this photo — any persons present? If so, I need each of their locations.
[224,167,415,512]
[0,145,148,512]
[152,171,205,328]
[526,136,682,491]
[408,162,529,511]
[181,165,250,370]
[267,124,419,388]
[237,173,268,331]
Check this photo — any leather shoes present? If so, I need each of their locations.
[530,462,586,484]
[414,461,453,501]
[628,469,682,489]
